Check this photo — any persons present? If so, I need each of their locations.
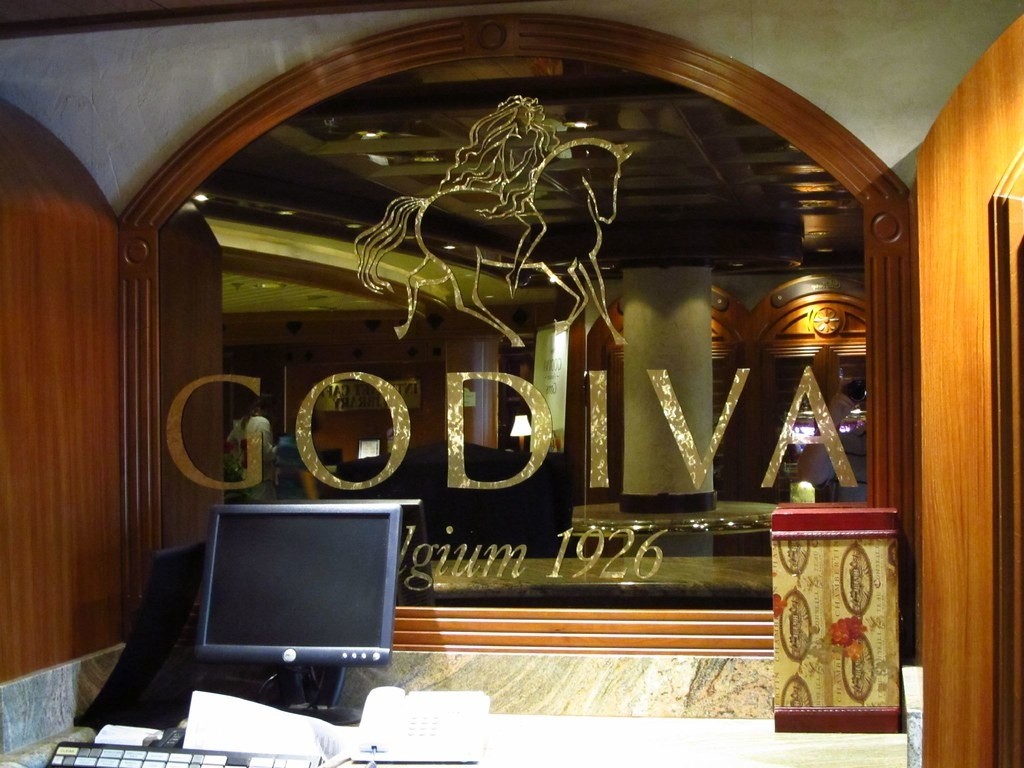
[798,380,868,503]
[226,405,320,501]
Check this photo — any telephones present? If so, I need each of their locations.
[351,684,492,764]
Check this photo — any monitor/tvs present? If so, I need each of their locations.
[304,500,439,606]
[194,502,404,725]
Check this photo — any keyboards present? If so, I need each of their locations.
[44,741,323,768]
